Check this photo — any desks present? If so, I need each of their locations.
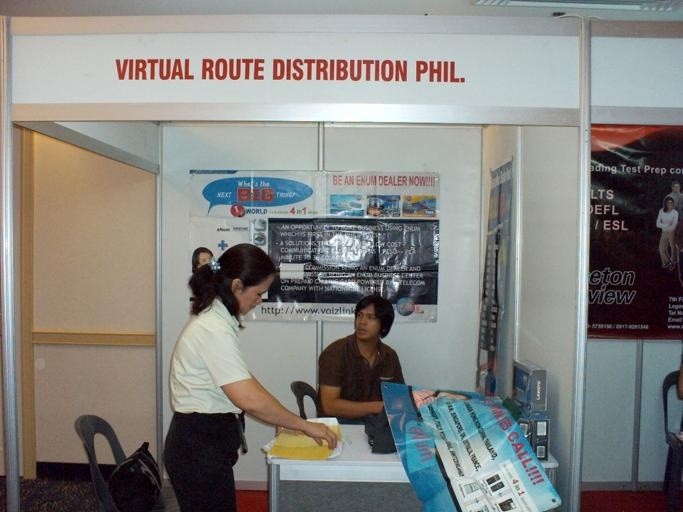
[266,417,560,512]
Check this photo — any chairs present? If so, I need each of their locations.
[289,378,325,418]
[72,411,183,512]
[659,366,682,512]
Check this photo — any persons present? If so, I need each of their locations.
[315,292,406,423]
[162,242,337,511]
[656,194,678,271]
[661,179,683,264]
[189,245,212,271]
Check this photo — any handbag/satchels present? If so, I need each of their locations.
[108,442,162,511]
[365,408,396,454]
